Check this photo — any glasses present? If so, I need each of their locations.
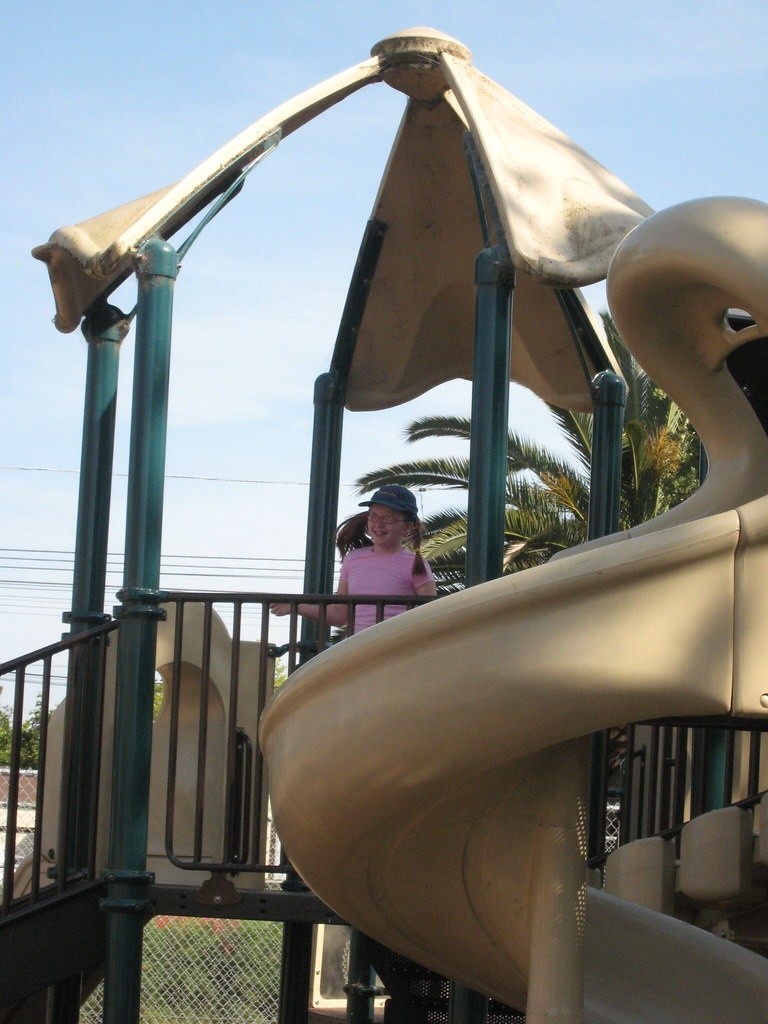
[367,514,409,523]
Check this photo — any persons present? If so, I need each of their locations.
[268,486,436,638]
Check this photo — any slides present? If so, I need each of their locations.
[258,483,768,1024]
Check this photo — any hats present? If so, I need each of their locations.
[358,486,418,514]
